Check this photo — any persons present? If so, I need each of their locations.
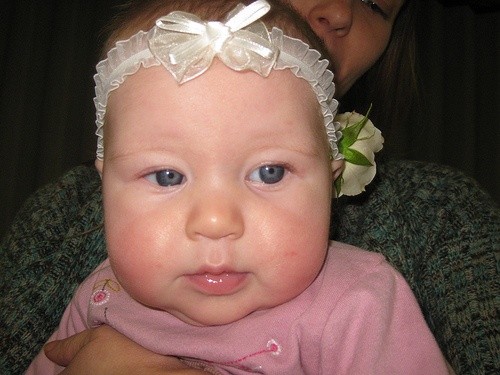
[20,0,456,375]
[0,0,500,375]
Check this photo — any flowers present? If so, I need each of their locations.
[334,103,384,198]
